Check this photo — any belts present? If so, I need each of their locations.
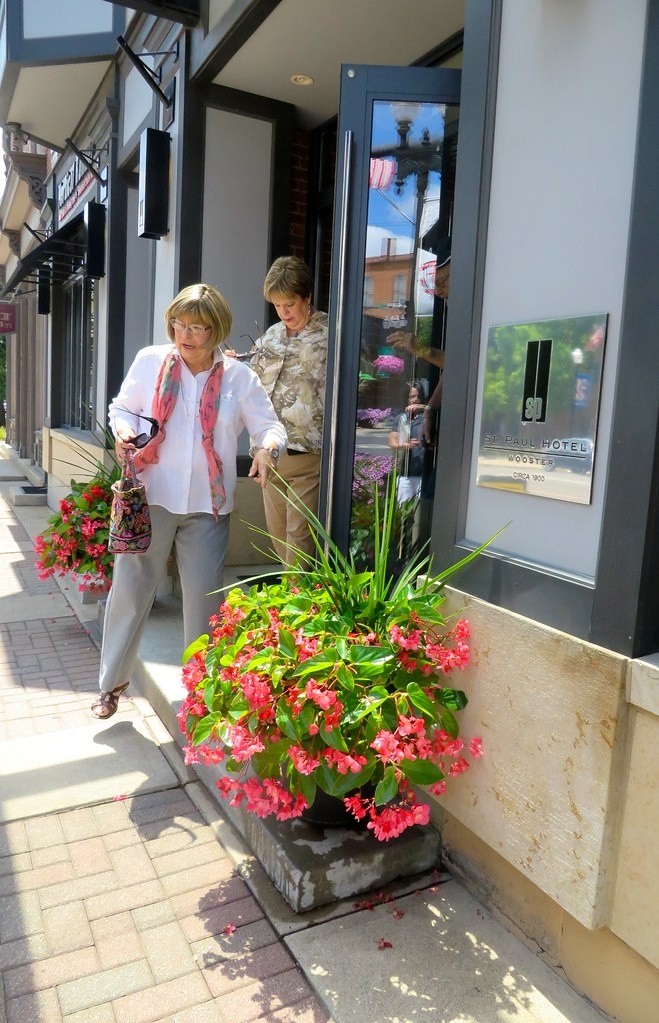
[287,448,308,456]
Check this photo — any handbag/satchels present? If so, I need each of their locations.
[108,450,151,554]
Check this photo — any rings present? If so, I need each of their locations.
[400,336,403,347]
[268,475,272,478]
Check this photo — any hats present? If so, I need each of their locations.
[419,215,454,269]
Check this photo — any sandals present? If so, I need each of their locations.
[91,680,130,719]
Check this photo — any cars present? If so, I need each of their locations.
[537,437,593,476]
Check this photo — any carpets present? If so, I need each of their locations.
[20,486,46,494]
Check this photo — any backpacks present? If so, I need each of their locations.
[408,410,427,477]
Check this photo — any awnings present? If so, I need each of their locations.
[0,210,87,299]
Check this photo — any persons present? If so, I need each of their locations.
[387,381,429,509]
[91,284,288,719]
[224,258,327,575]
[386,235,452,571]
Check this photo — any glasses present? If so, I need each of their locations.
[168,318,212,335]
[432,274,450,288]
[222,333,262,359]
[112,407,159,449]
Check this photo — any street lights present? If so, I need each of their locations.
[367,100,443,421]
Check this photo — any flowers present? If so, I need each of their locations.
[176,457,515,843]
[34,399,122,595]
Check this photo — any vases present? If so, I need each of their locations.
[295,781,376,827]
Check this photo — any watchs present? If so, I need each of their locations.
[261,447,279,461]
